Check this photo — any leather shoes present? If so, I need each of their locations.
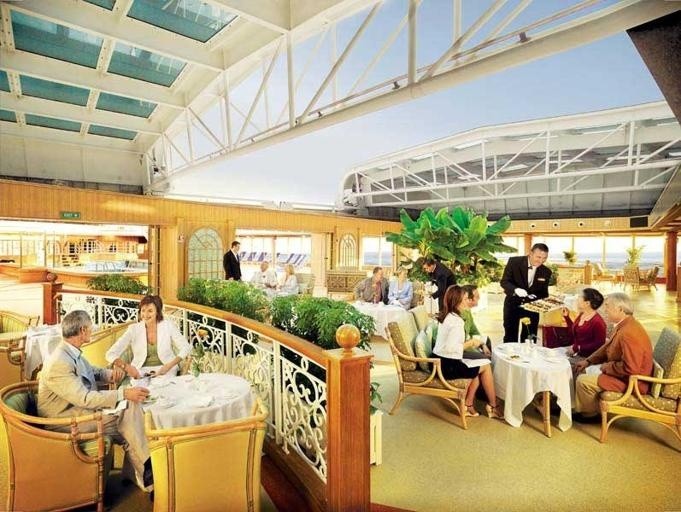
[143,470,153,487]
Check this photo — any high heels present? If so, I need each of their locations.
[464,405,479,417]
[486,403,504,419]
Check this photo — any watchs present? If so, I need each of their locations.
[120,361,127,369]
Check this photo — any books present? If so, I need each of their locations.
[519,294,569,315]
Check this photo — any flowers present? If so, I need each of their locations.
[520,316,540,342]
[187,326,211,376]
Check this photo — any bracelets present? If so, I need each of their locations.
[471,341,475,347]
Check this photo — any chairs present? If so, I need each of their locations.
[386,275,414,309]
[3,310,42,358]
[599,326,681,449]
[384,305,473,430]
[2,378,103,511]
[143,385,268,512]
[79,330,116,367]
[594,260,661,294]
[110,319,139,366]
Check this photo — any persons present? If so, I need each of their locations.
[562,287,608,368]
[37,311,154,488]
[433,285,493,364]
[500,243,552,343]
[221,242,241,281]
[388,267,414,310]
[424,256,457,312]
[105,296,190,380]
[274,265,298,295]
[249,258,278,290]
[571,293,653,423]
[434,284,504,420]
[355,266,390,304]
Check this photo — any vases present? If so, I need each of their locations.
[523,338,538,356]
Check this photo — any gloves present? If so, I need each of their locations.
[514,288,528,298]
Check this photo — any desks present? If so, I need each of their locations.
[117,373,259,494]
[494,341,577,439]
[353,300,404,341]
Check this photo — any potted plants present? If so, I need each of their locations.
[562,249,579,266]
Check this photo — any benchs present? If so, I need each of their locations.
[275,264,392,299]
